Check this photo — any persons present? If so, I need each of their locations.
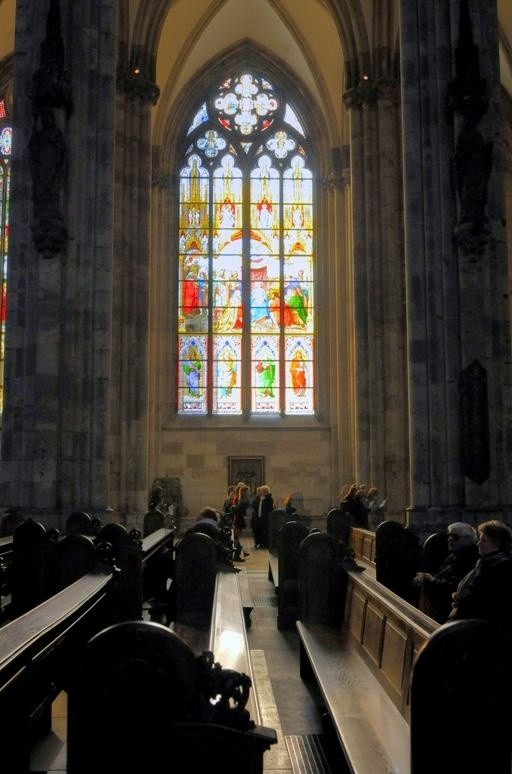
[286,494,296,515]
[257,350,276,396]
[290,351,308,395]
[415,522,480,622]
[224,483,273,556]
[449,520,510,621]
[218,349,237,396]
[180,268,309,326]
[346,484,384,529]
[197,508,225,522]
[182,349,202,398]
[148,485,166,520]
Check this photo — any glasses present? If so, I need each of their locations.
[445,533,470,542]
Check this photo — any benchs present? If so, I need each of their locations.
[0,504,512,774]
